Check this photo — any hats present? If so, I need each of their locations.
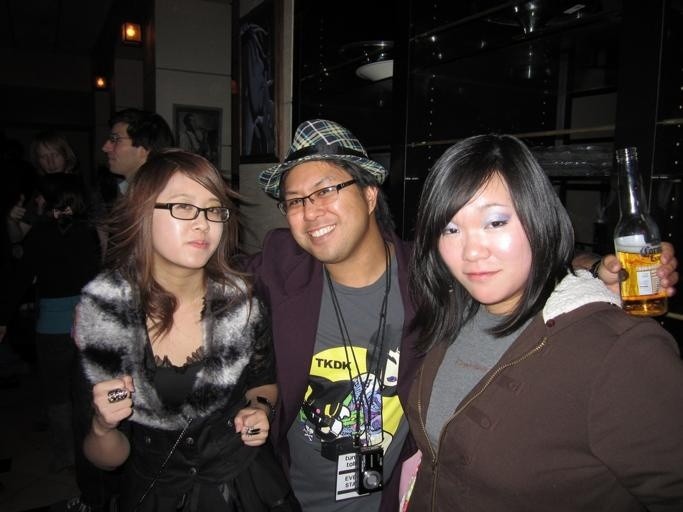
[258,119,389,200]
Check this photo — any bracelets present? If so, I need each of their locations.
[239,397,274,413]
[589,260,601,278]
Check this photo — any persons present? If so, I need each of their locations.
[394,132,682,509]
[32,107,175,512]
[19,172,106,470]
[78,148,281,511]
[8,137,93,247]
[232,119,681,510]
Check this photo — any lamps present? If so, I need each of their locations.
[119,21,144,49]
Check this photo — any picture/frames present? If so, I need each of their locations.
[172,103,223,172]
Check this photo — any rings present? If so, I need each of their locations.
[107,387,127,402]
[247,429,261,435]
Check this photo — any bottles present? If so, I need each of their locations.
[610,144,670,317]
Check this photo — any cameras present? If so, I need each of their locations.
[355,446,383,495]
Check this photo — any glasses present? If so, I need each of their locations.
[277,179,357,216]
[110,133,131,142]
[154,203,231,222]
